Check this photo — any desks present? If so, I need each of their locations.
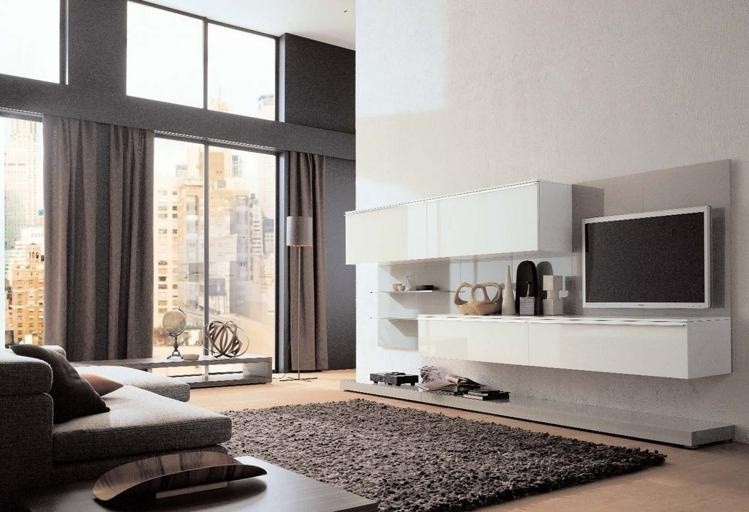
[1,454,378,511]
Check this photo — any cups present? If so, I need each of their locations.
[392,283,405,292]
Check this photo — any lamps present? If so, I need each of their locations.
[285,215,315,381]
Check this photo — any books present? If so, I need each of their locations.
[420,368,509,402]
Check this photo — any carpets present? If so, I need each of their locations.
[216,397,664,511]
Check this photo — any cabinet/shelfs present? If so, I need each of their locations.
[344,181,573,264]
[415,312,732,380]
[68,354,272,390]
[368,286,451,320]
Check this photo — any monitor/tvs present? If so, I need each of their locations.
[582,204,712,309]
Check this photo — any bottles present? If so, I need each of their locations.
[502,265,515,315]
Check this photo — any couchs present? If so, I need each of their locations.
[1,345,231,511]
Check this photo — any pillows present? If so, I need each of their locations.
[5,342,124,425]
